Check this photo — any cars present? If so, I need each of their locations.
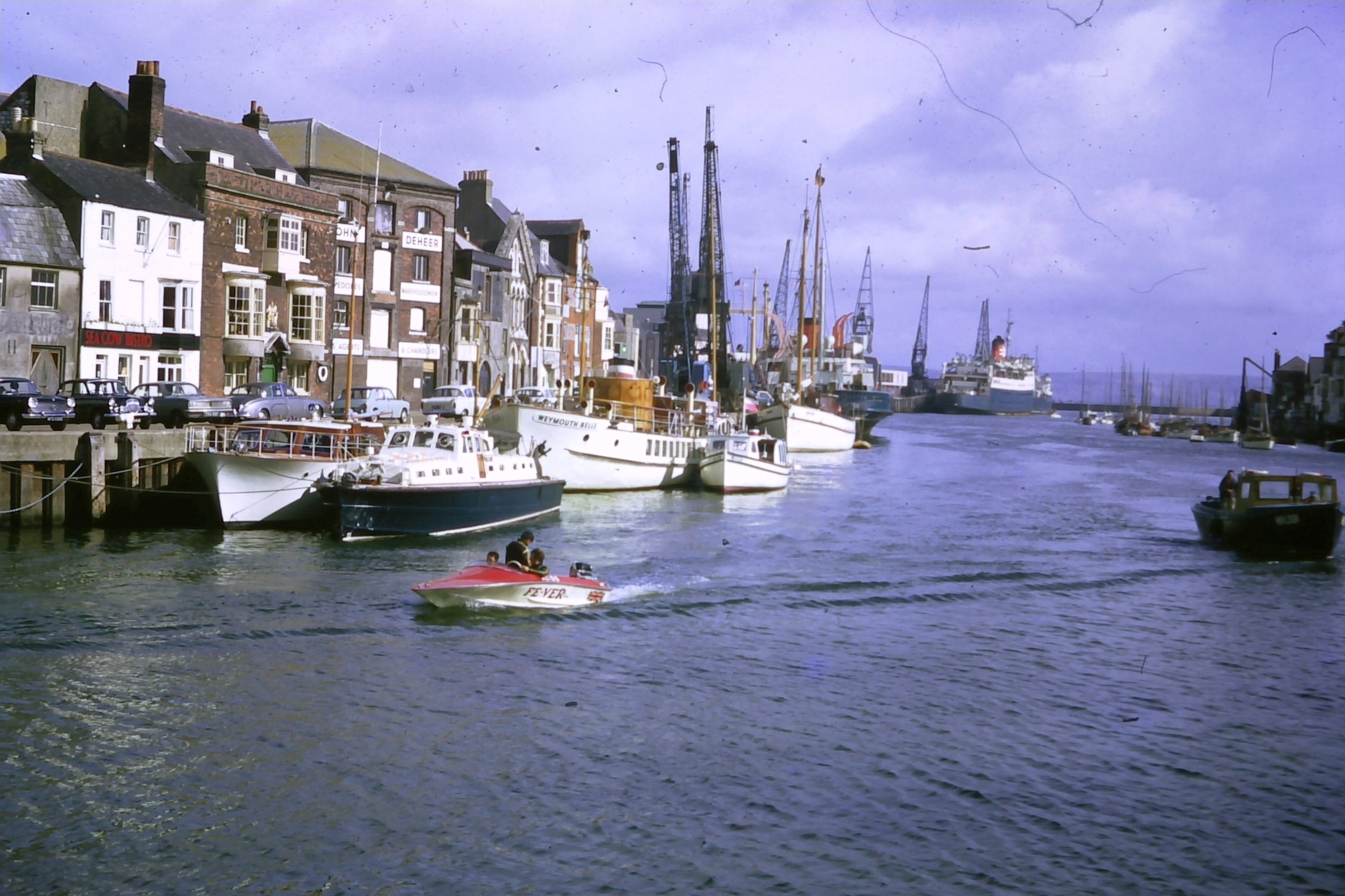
[229,382,329,421]
[511,388,558,410]
[130,381,232,429]
[421,385,491,417]
[0,377,77,431]
[333,387,411,423]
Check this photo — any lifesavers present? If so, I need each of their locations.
[317,365,329,382]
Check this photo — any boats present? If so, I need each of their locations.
[410,561,612,611]
[1191,467,1344,561]
[181,229,567,542]
[1325,438,1345,452]
[480,258,793,494]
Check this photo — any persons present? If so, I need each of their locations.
[487,551,499,563]
[506,531,549,577]
[372,475,382,486]
[1218,470,1237,508]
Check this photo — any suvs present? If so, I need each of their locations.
[55,378,158,430]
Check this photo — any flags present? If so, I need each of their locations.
[814,168,825,187]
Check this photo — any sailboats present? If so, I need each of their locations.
[937,297,1053,416]
[1074,355,1276,450]
[654,107,946,453]
